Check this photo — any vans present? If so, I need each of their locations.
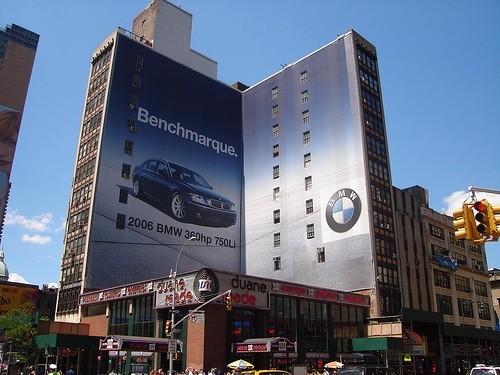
[335,353,385,375]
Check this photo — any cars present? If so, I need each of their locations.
[238,367,293,375]
[465,363,500,375]
[131,156,237,227]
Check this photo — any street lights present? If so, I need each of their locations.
[168,237,197,375]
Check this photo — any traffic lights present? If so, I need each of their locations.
[452,204,474,241]
[487,202,500,238]
[225,296,232,311]
[165,320,172,336]
[473,198,490,236]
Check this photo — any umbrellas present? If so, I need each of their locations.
[324,360,344,369]
[227,359,255,369]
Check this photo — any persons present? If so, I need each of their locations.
[28,366,36,375]
[108,370,115,375]
[323,369,329,375]
[149,367,165,375]
[66,365,75,375]
[175,367,235,375]
